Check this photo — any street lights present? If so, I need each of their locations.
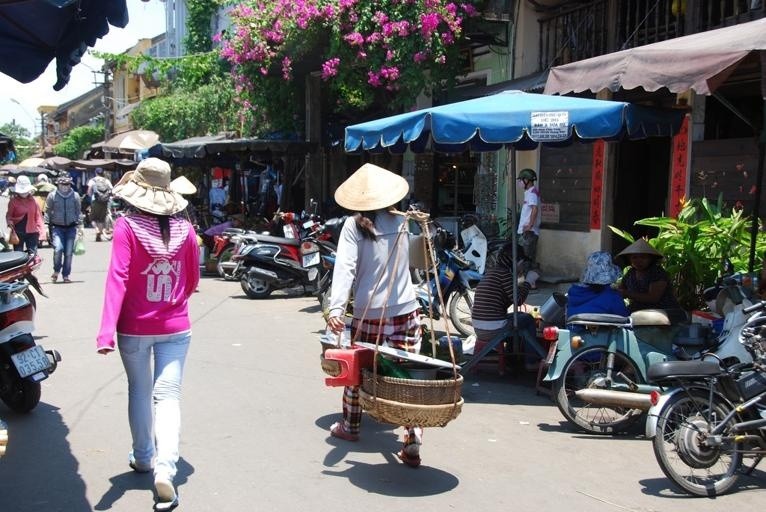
[9,98,37,139]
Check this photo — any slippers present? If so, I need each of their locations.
[330,421,361,441]
[397,448,421,466]
[154,494,179,511]
[126,448,155,473]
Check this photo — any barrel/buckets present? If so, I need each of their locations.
[400,362,444,380]
[435,215,461,239]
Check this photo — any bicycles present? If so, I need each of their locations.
[98,197,128,241]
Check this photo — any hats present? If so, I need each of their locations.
[228,213,246,224]
[10,175,38,194]
[37,173,49,182]
[613,237,664,269]
[170,175,197,195]
[0,176,17,188]
[95,167,104,174]
[110,157,188,216]
[334,163,410,212]
[54,170,75,185]
[579,252,622,286]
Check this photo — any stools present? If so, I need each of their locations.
[472,337,525,380]
[534,356,586,404]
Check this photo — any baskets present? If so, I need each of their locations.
[320,340,351,377]
[355,361,464,428]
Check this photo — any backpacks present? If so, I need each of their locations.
[91,177,112,202]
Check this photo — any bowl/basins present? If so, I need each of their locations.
[401,361,440,380]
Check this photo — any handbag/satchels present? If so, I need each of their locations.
[7,225,20,245]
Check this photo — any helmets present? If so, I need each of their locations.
[516,169,538,181]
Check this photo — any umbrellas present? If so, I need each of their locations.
[344,89,692,388]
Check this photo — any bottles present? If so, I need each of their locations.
[375,353,410,379]
[530,307,539,334]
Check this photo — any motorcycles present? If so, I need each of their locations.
[319,216,484,336]
[644,299,766,498]
[301,215,346,305]
[209,206,301,282]
[0,238,63,412]
[222,198,329,297]
[544,261,766,432]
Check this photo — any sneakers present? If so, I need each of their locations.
[50,275,57,281]
[96,232,101,242]
[64,279,71,283]
